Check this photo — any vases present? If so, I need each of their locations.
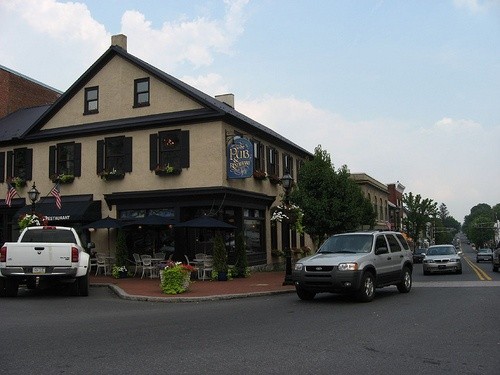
[254,175,266,180]
[159,270,191,293]
[270,177,281,184]
[10,170,181,187]
[278,210,299,223]
[28,221,43,226]
[118,270,126,278]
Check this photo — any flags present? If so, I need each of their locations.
[5,184,17,208]
[50,181,62,210]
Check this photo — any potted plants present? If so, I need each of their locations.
[211,227,249,283]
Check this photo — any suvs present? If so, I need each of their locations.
[491,240,500,272]
[293,229,414,304]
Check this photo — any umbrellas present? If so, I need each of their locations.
[120,214,179,256]
[81,215,128,263]
[172,215,236,255]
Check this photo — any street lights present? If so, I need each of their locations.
[280,171,296,286]
[27,181,40,217]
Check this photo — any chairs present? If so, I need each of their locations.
[432,250,438,254]
[184,253,213,281]
[444,249,452,254]
[132,252,173,279]
[89,253,114,276]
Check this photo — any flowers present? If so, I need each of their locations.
[112,265,127,278]
[270,204,304,234]
[160,262,194,294]
[253,171,266,176]
[5,163,181,183]
[271,175,279,180]
[18,214,44,228]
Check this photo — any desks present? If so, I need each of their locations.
[143,258,165,278]
[193,258,214,281]
[98,256,112,275]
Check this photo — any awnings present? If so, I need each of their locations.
[14,198,102,224]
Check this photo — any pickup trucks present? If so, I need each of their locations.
[0,226,96,297]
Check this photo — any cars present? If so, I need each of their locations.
[412,248,429,264]
[421,244,464,275]
[455,246,463,255]
[476,249,494,263]
